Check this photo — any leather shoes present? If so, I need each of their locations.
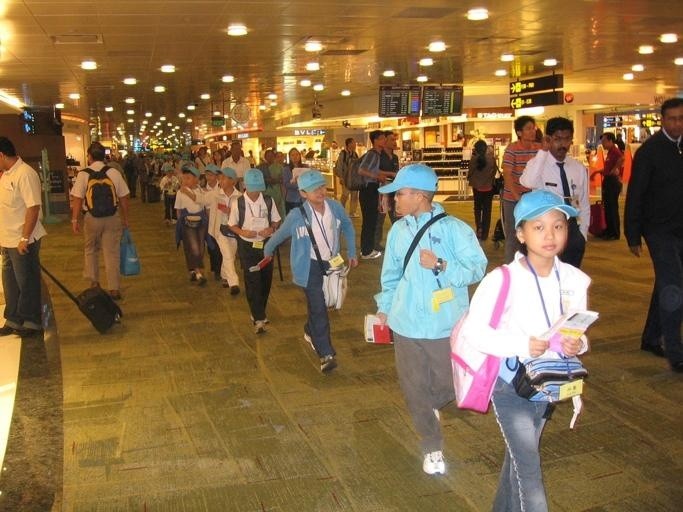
[595,229,620,241]
[641,339,682,374]
[90,281,100,288]
[111,289,121,300]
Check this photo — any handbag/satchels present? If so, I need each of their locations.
[510,354,589,403]
[120,226,140,276]
[320,265,352,311]
[184,215,203,229]
[219,223,238,237]
[492,163,504,194]
[446,265,511,414]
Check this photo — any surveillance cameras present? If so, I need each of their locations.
[312,112,321,119]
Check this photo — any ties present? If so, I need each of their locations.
[556,161,573,206]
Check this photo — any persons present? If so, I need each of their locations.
[461,190,592,510]
[1,136,47,338]
[372,164,488,476]
[334,130,404,258]
[123,140,360,372]
[468,115,623,268]
[622,99,683,371]
[69,142,131,297]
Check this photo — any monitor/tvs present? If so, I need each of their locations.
[378,85,422,118]
[422,86,463,118]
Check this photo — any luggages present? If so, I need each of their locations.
[147,180,161,203]
[75,286,123,337]
[589,197,608,235]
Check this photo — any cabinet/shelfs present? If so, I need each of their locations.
[421,147,462,183]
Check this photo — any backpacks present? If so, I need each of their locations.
[345,149,379,192]
[331,150,346,178]
[78,166,119,218]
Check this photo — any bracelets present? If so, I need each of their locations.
[432,257,443,276]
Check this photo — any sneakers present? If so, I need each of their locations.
[359,248,382,260]
[189,270,207,287]
[0,325,46,341]
[302,332,317,354]
[476,223,483,239]
[320,356,338,372]
[249,313,271,334]
[422,450,446,477]
[349,212,360,218]
[220,275,240,296]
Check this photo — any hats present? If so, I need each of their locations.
[513,188,580,231]
[164,165,175,172]
[203,163,220,175]
[243,168,267,194]
[296,168,328,194]
[181,165,201,179]
[377,163,440,196]
[215,167,238,180]
